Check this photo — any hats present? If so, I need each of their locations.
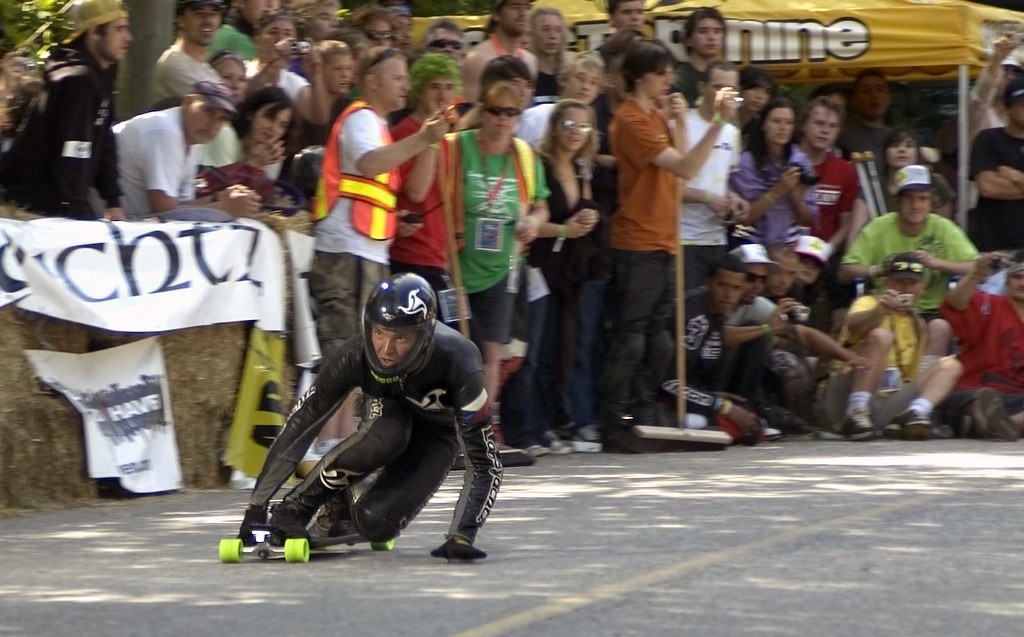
[60,0,128,44]
[1001,46,1024,69]
[189,82,237,114]
[180,0,222,9]
[728,244,778,265]
[1005,78,1024,105]
[889,254,924,281]
[895,165,932,196]
[792,236,831,263]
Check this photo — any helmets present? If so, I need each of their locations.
[362,272,437,383]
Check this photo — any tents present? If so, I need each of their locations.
[410,1,1024,229]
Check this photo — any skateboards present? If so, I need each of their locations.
[217,532,401,564]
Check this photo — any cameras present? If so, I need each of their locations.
[730,92,744,108]
[993,256,1011,268]
[18,57,37,74]
[895,293,914,307]
[787,303,811,321]
[290,41,311,54]
[791,162,821,186]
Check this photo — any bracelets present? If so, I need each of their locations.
[868,265,876,276]
[705,192,714,202]
[719,401,734,415]
[429,143,439,149]
[764,194,776,204]
[553,225,566,251]
[762,324,770,334]
[713,116,727,125]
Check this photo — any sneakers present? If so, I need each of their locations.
[266,503,310,551]
[315,486,357,536]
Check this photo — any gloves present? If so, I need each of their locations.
[236,503,268,546]
[431,536,486,559]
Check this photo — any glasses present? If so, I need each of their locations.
[742,271,768,285]
[365,48,394,70]
[555,120,594,132]
[892,262,924,273]
[363,30,393,40]
[427,39,462,49]
[796,254,819,267]
[483,106,521,117]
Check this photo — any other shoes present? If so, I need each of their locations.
[492,389,1022,456]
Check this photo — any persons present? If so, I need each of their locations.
[446,86,553,468]
[508,101,601,455]
[312,47,448,461]
[211,49,249,105]
[515,0,1024,456]
[233,86,294,180]
[208,0,282,60]
[0,0,134,222]
[389,52,461,332]
[245,9,330,125]
[90,80,263,220]
[464,0,539,102]
[301,40,355,149]
[235,273,503,560]
[145,0,245,167]
[290,0,466,125]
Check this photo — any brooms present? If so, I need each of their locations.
[628,115,734,454]
[433,142,538,471]
